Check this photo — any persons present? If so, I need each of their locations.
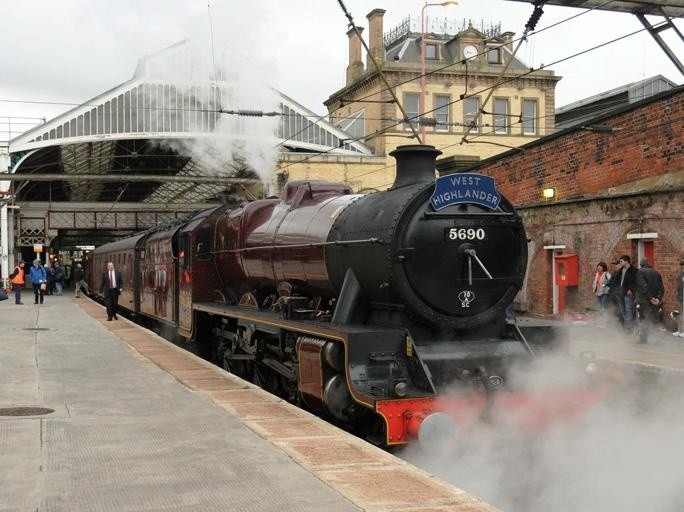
[8,261,26,305]
[671,259,684,339]
[592,254,666,346]
[29,259,65,305]
[73,263,92,299]
[98,260,124,321]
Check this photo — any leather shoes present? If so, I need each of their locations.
[107,316,118,321]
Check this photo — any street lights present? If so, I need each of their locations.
[420,1,459,143]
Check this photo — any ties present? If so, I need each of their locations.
[109,270,113,288]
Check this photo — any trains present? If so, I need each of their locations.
[72,145,608,454]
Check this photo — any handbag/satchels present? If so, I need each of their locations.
[40,283,47,290]
